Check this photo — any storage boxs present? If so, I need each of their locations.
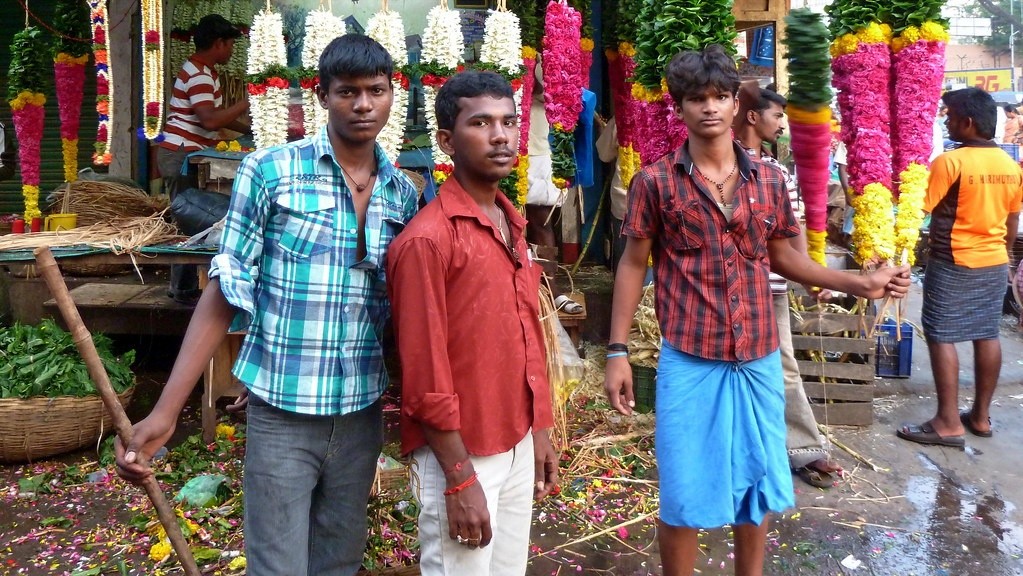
[630,363,657,415]
[862,315,913,377]
[998,143,1019,162]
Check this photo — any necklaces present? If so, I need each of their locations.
[337,159,375,192]
[493,202,510,248]
[701,159,737,207]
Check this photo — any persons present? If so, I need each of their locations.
[739,87,841,488]
[387,70,558,576]
[897,87,1023,447]
[515,52,584,314]
[595,110,629,280]
[114,34,418,576]
[605,43,912,576]
[156,14,251,305]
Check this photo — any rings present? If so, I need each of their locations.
[468,537,480,546]
[457,535,468,545]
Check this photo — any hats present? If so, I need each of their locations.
[198,14,242,39]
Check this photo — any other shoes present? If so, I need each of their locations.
[168,289,202,305]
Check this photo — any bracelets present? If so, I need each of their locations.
[442,453,477,495]
[606,342,628,357]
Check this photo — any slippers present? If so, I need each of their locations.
[897,421,965,447]
[960,409,992,436]
[553,294,585,314]
[799,463,836,488]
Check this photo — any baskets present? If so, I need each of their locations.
[49,179,172,237]
[0,357,137,462]
